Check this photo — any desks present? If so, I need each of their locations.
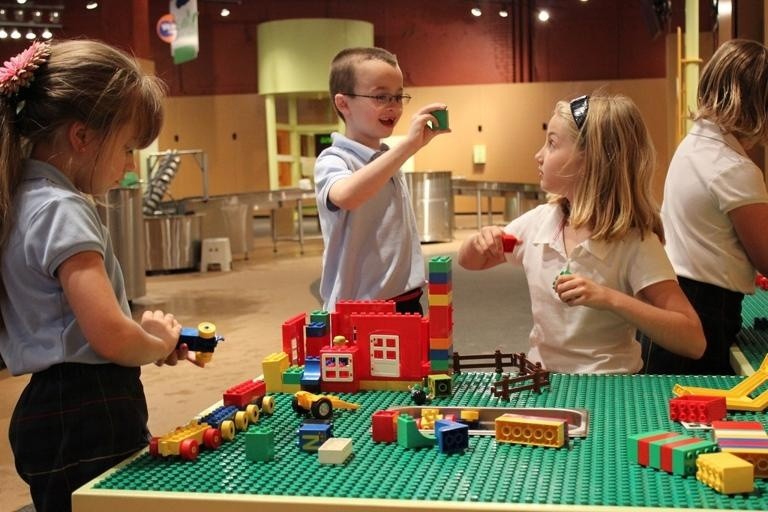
[71,370,767,509]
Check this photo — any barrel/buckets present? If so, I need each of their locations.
[401,170,456,245]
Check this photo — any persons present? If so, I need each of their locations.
[658,39,768,375]
[457,93,707,375]
[314,45,452,314]
[0,40,205,512]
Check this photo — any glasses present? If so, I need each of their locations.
[344,92,412,106]
